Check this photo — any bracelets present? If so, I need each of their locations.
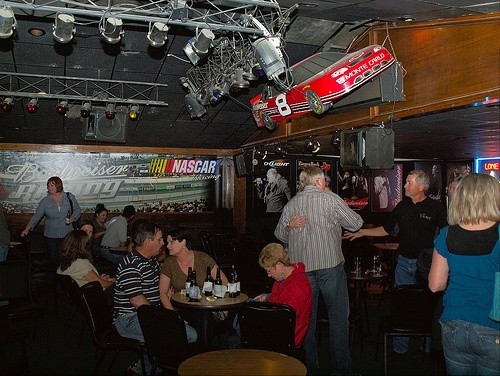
[286,220,290,228]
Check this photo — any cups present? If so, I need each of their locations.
[372,255,380,274]
[353,256,362,273]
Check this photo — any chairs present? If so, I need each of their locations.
[0,223,448,376]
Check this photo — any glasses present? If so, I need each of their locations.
[267,262,278,274]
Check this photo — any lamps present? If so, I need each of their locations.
[0,6,287,119]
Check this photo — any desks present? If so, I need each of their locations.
[168,289,249,353]
[177,348,308,376]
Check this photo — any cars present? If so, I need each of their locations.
[249,44,397,132]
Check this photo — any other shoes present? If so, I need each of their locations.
[411,349,426,359]
[387,350,402,363]
[128,360,152,376]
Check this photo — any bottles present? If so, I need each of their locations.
[65,210,70,225]
[186,264,240,303]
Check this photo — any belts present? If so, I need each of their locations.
[100,246,112,248]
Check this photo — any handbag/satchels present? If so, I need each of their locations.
[73,217,81,228]
[488,272,500,322]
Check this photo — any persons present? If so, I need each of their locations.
[428,175,500,376]
[75,219,94,245]
[56,230,115,309]
[138,196,213,212]
[275,165,365,376]
[430,166,440,199]
[254,169,291,212]
[159,225,230,353]
[92,204,108,256]
[228,242,312,348]
[337,169,368,200]
[111,220,197,376]
[22,178,82,268]
[344,170,447,363]
[374,171,392,211]
[101,205,135,256]
[450,176,464,197]
[0,201,11,306]
